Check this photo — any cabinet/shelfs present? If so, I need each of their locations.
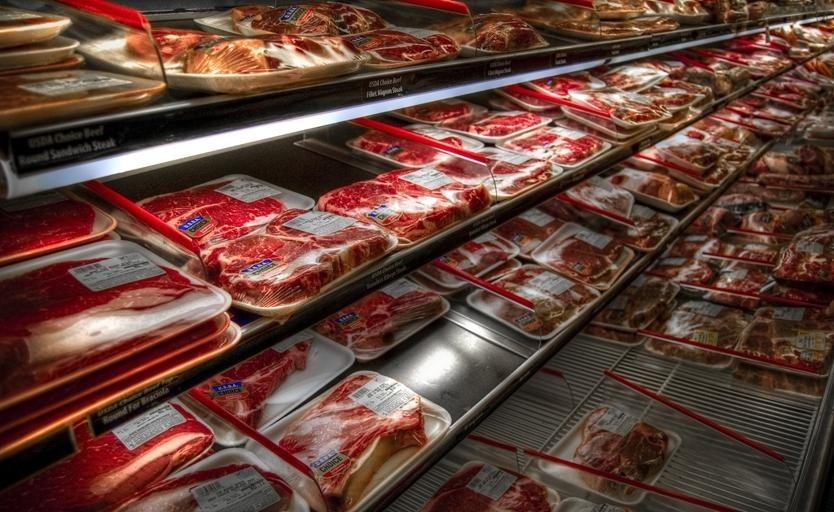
[0,1,833,511]
